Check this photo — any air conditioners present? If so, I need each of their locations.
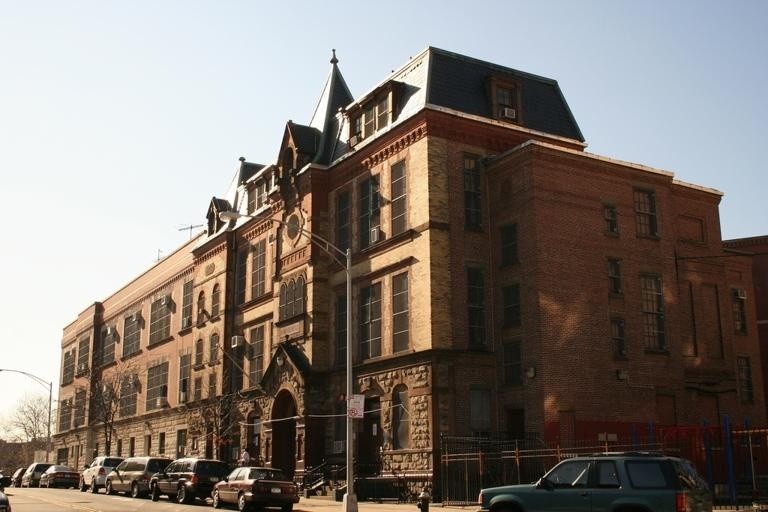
[733,287,747,299]
[178,392,186,404]
[78,362,87,374]
[231,335,245,350]
[371,227,381,244]
[106,295,170,335]
[501,107,516,120]
[156,395,167,409]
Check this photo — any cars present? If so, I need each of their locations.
[11,468,27,488]
[39,464,80,489]
[211,466,300,511]
[0,491,12,512]
[0,473,13,490]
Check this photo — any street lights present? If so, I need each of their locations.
[0,368,53,463]
[215,211,358,512]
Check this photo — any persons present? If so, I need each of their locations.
[240,448,250,466]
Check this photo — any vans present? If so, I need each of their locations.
[105,457,176,498]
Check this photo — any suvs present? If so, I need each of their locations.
[20,462,55,488]
[477,450,715,512]
[79,457,126,493]
[148,458,229,505]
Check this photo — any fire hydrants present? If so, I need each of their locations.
[416,486,431,512]
